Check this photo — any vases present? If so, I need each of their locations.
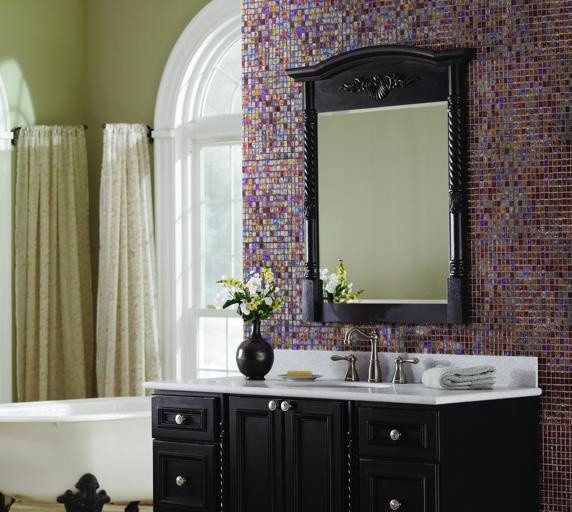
[234,315,274,384]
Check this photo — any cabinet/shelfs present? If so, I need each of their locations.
[151,389,545,512]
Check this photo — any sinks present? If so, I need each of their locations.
[279,380,393,389]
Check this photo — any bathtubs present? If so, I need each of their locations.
[0,396,156,512]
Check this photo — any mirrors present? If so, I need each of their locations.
[286,44,482,326]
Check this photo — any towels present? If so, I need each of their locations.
[421,359,499,395]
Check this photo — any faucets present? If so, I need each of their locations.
[343,327,383,382]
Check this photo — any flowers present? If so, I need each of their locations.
[312,254,365,307]
[210,261,289,320]
